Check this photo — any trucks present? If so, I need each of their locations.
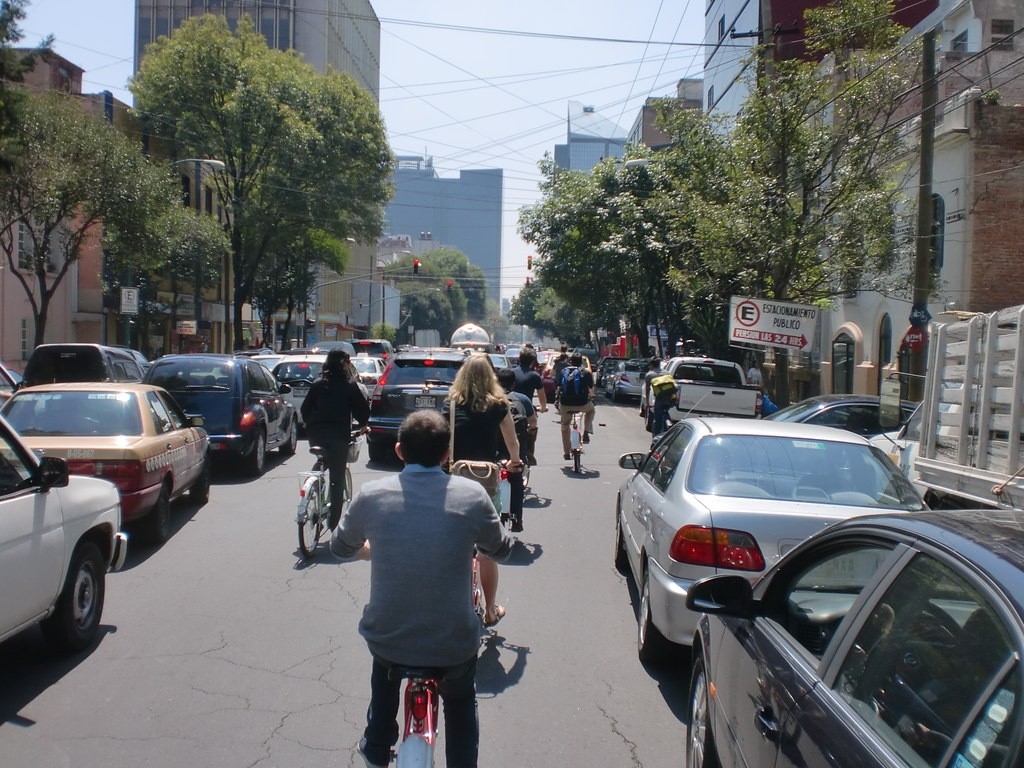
[869,304,1024,510]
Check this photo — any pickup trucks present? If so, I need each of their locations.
[638,357,762,423]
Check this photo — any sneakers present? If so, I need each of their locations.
[357,737,388,768]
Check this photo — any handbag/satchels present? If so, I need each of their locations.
[762,394,776,412]
[451,460,499,491]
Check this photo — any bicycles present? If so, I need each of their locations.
[567,411,588,473]
[471,407,548,615]
[388,663,440,768]
[295,432,367,557]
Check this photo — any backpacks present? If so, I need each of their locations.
[561,367,587,403]
[505,392,527,439]
[649,374,678,397]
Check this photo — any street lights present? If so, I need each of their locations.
[368,251,411,338]
[297,237,355,348]
[625,159,766,375]
[122,159,225,349]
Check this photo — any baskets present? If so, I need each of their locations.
[346,437,363,463]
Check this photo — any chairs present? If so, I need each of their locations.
[30,396,137,432]
[701,443,875,502]
[172,375,228,385]
[927,607,1009,731]
[407,367,458,383]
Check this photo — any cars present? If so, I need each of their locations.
[0,382,212,547]
[143,353,299,477]
[686,509,1024,768]
[762,393,920,439]
[366,323,599,462]
[22,337,418,424]
[596,357,649,402]
[615,417,930,675]
[0,363,130,652]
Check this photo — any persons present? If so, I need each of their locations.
[440,355,520,625]
[762,384,779,418]
[299,347,370,531]
[747,360,763,384]
[496,368,536,532]
[556,353,596,460]
[332,409,514,768]
[645,357,677,449]
[553,345,570,406]
[510,349,546,465]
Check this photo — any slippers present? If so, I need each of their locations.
[484,605,505,626]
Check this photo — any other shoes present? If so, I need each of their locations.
[583,434,589,442]
[512,518,524,532]
[564,454,570,460]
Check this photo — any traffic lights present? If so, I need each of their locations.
[528,256,531,270]
[414,259,419,274]
[447,280,451,292]
